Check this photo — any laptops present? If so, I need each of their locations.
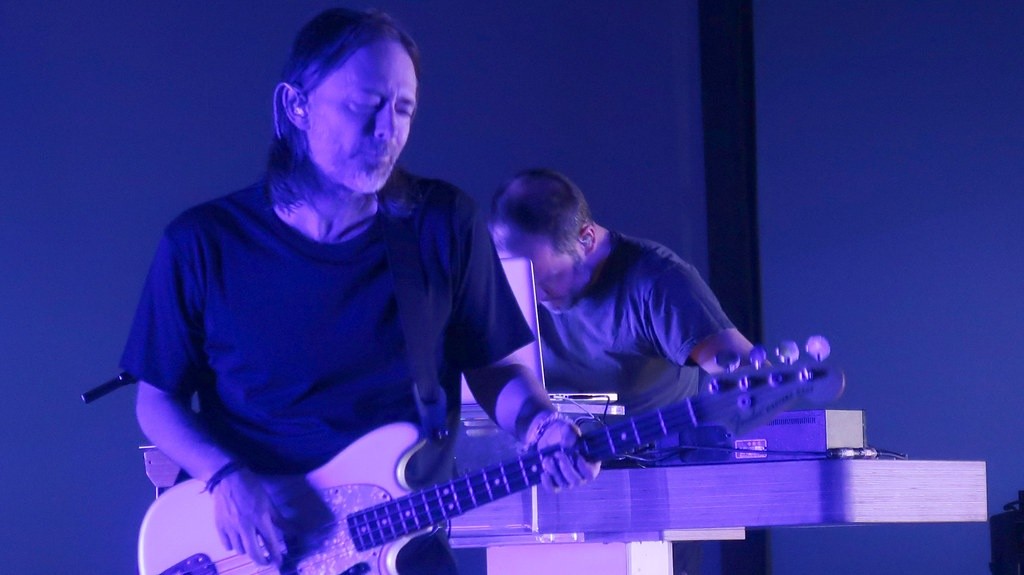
[461,256,618,405]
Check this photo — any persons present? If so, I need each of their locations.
[119,6,601,575]
[486,167,775,427]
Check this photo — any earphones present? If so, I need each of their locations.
[293,100,308,118]
[582,236,593,249]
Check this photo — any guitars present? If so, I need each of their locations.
[139,331,845,575]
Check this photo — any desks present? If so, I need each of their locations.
[138,443,988,575]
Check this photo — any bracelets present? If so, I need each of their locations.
[201,459,249,495]
[525,413,581,453]
